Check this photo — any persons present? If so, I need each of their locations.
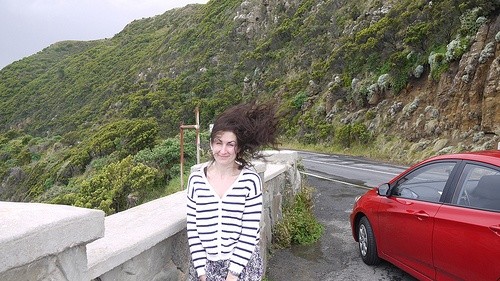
[185,98,283,280]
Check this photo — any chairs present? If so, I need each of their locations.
[461,175,500,211]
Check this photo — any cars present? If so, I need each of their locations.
[349,149,500,281]
